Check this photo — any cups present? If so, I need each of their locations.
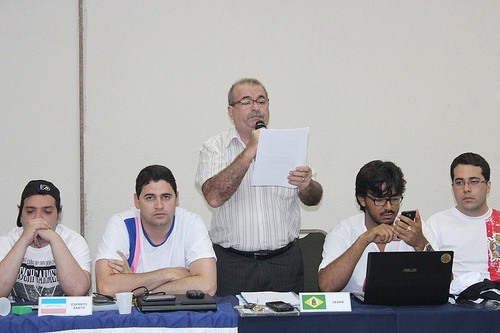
[0,297,11,317]
[115,292,134,314]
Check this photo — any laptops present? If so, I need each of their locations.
[351,250,454,306]
[137,293,217,311]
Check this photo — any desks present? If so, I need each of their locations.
[0,295,500,333]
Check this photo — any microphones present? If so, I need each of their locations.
[256,120,266,130]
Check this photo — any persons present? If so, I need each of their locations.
[0,180,92,302]
[319,159,453,294]
[96,165,217,296]
[195,78,323,295]
[425,152,500,294]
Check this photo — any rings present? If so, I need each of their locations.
[303,178,304,181]
[407,226,411,231]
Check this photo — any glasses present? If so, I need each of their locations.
[453,179,488,185]
[230,95,269,107]
[366,195,403,206]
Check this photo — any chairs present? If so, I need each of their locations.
[298,229,328,292]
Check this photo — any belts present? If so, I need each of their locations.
[227,238,297,259]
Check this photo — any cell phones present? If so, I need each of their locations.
[186,290,205,298]
[266,301,294,312]
[400,210,417,225]
[143,295,176,302]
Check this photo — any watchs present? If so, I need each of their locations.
[423,243,433,251]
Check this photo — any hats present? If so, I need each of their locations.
[16,180,60,227]
